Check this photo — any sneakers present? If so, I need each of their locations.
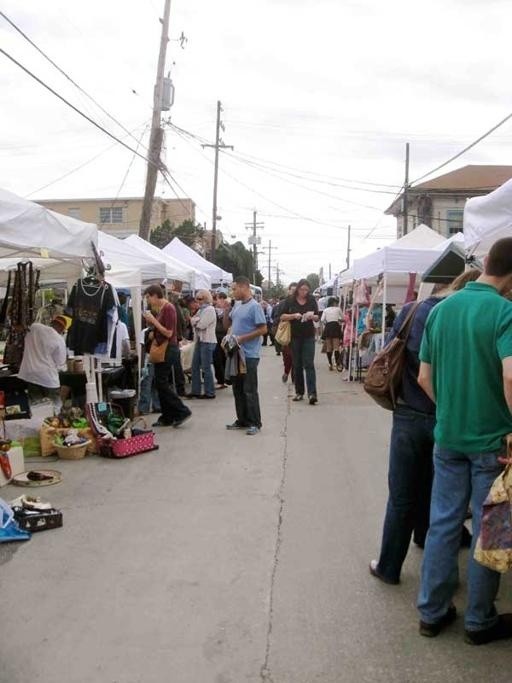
[226,421,246,430]
[368,558,400,584]
[246,426,260,435]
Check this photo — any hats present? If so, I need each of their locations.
[56,315,73,329]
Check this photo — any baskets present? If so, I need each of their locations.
[39,416,96,460]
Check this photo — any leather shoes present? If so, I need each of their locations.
[172,409,192,427]
[419,603,456,637]
[151,419,166,426]
[197,393,215,398]
[464,612,512,646]
[309,395,318,404]
[293,393,303,400]
[283,373,288,381]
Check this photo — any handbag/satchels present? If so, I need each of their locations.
[0,497,32,542]
[366,286,383,332]
[473,433,512,574]
[147,339,168,364]
[275,320,292,346]
[363,301,421,410]
[355,278,370,305]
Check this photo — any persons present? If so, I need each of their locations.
[223,276,268,433]
[370,269,481,586]
[416,235,512,646]
[15,274,343,427]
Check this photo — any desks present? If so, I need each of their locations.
[0,349,137,412]
[166,341,204,395]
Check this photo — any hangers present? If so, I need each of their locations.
[73,267,108,289]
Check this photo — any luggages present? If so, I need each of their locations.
[84,401,159,458]
